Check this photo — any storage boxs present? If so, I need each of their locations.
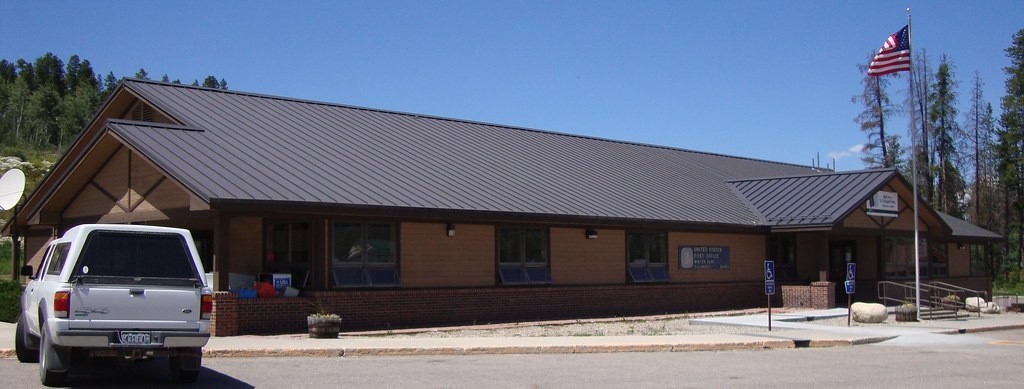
[229,272,299,298]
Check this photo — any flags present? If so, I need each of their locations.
[867,25,910,76]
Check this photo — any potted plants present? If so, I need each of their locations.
[941,295,960,311]
[307,299,343,339]
[895,296,917,321]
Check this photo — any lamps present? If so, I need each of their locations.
[447,223,456,237]
[586,230,599,239]
[957,244,967,250]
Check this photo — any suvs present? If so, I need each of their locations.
[15,223,215,386]
